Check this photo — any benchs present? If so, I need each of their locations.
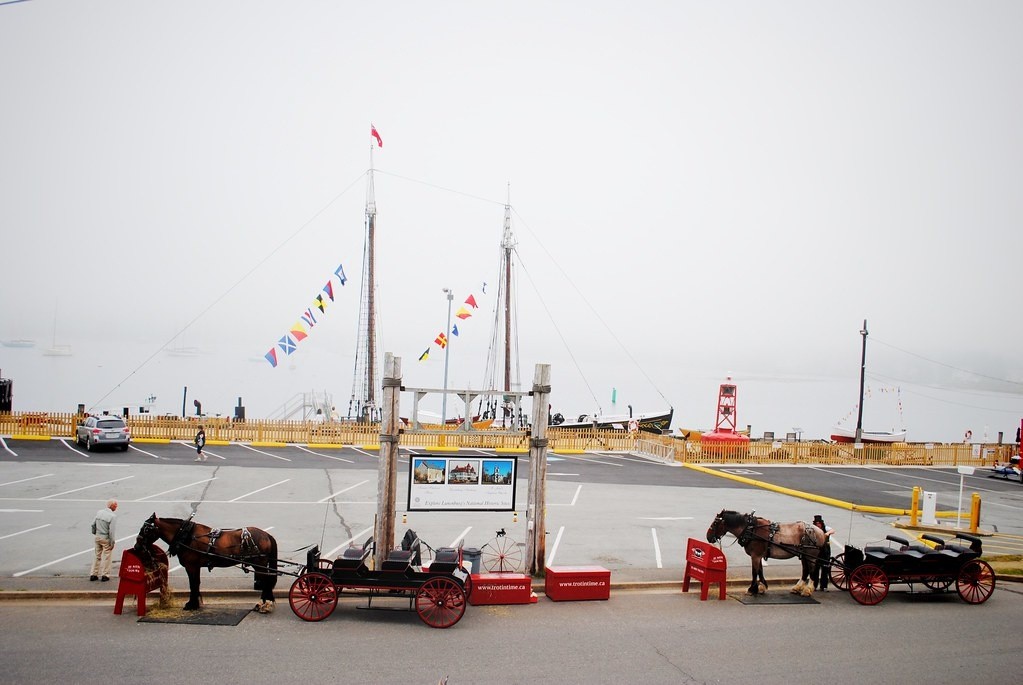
[864,533,982,561]
[382,538,420,570]
[428,539,465,572]
[332,536,373,568]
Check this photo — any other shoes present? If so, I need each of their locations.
[195,456,208,462]
[89,575,99,582]
[813,587,829,592]
[100,575,110,581]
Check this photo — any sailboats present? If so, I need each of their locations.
[309,116,674,436]
[830,319,907,444]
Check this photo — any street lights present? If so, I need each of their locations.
[441,288,454,425]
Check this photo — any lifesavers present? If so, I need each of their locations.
[628,420,638,431]
[964,430,972,439]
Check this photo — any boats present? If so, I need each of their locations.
[678,424,753,447]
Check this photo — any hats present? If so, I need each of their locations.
[813,515,824,522]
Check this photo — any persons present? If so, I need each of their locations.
[330,407,339,420]
[813,515,835,591]
[194,425,208,462]
[90,497,117,582]
[316,408,325,424]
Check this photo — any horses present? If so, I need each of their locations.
[706,508,834,597]
[135,512,278,614]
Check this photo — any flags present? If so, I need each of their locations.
[372,124,382,148]
[289,320,308,341]
[277,333,297,355]
[265,347,278,367]
[456,306,472,320]
[322,280,334,302]
[301,307,317,327]
[838,385,904,429]
[452,323,459,336]
[419,346,430,362]
[312,294,327,313]
[334,263,347,286]
[482,281,488,296]
[434,332,447,348]
[465,294,479,309]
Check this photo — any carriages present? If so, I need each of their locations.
[707,508,996,607]
[134,511,484,629]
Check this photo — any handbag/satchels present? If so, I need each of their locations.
[92,524,96,535]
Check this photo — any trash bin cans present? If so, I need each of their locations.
[921,491,938,525]
[236,407,245,422]
[462,547,483,574]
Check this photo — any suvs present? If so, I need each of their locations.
[75,415,131,451]
